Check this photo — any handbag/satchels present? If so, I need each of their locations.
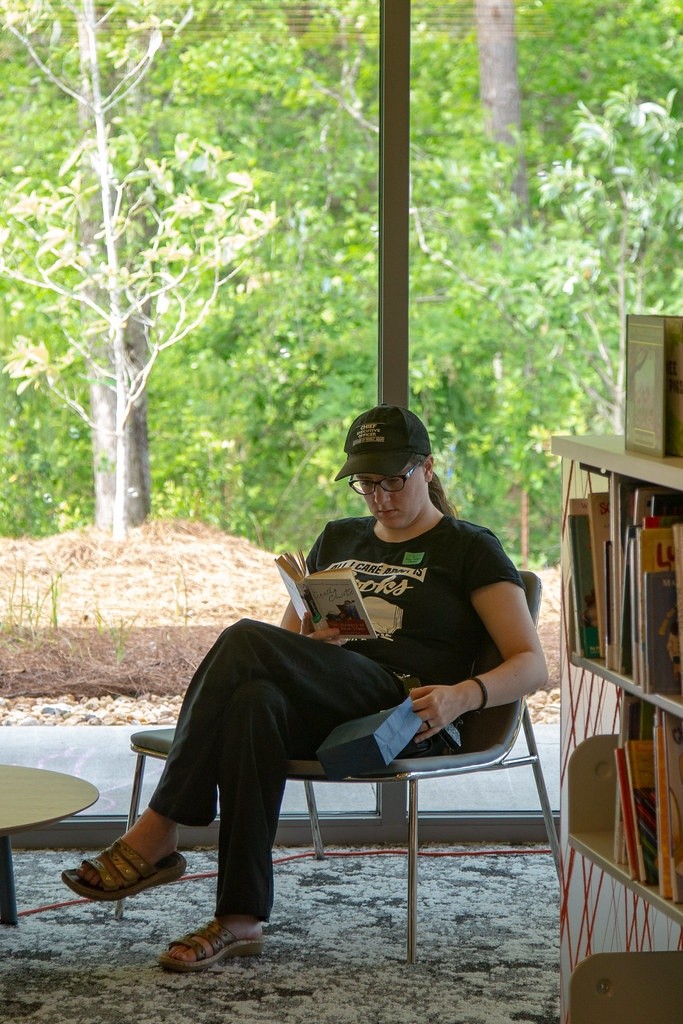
[315,696,424,780]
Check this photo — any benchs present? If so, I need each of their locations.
[113,569,563,966]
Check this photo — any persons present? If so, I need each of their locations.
[61,403,547,973]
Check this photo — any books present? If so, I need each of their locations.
[570,470,683,905]
[626,314,683,459]
[275,549,378,640]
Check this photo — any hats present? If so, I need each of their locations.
[334,404,432,482]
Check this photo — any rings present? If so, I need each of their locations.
[425,721,431,729]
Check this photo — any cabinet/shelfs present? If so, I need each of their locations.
[551,435,683,1024]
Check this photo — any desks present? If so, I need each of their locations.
[0,764,99,930]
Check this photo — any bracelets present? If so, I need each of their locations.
[468,677,488,712]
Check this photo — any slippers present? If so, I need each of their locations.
[159,920,263,972]
[61,837,187,901]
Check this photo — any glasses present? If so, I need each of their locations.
[348,461,421,496]
